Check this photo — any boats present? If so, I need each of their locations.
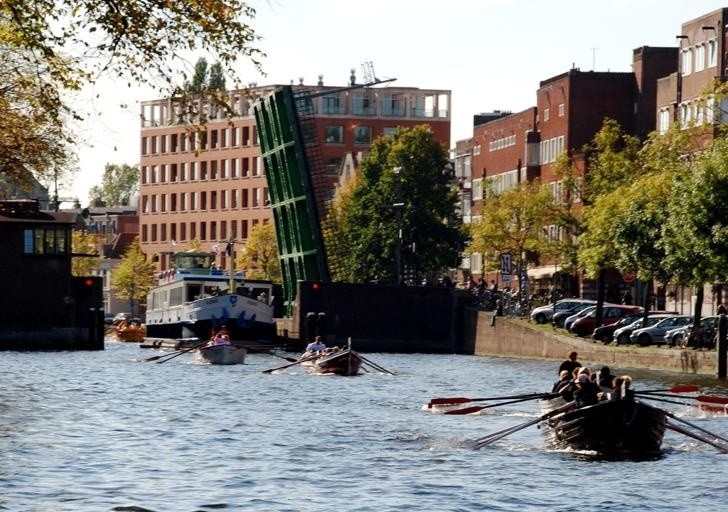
[538,391,668,463]
[299,336,362,376]
[117,228,278,365]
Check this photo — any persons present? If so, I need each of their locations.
[464,273,498,297]
[623,291,634,305]
[557,352,582,374]
[552,366,635,408]
[306,335,327,353]
[205,324,231,347]
[488,298,503,327]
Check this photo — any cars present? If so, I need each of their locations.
[530,297,728,350]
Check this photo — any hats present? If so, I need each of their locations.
[578,366,589,375]
[574,373,588,384]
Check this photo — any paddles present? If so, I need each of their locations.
[443,398,535,414]
[644,392,728,406]
[146,346,189,362]
[261,357,319,373]
[636,394,727,415]
[634,384,701,393]
[428,397,553,408]
[246,348,298,362]
[140,345,193,349]
